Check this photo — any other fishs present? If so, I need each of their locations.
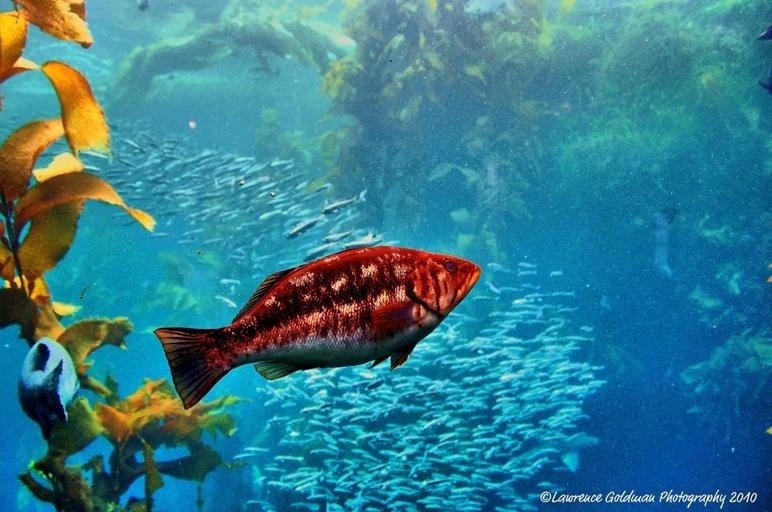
[14,119,615,512]
